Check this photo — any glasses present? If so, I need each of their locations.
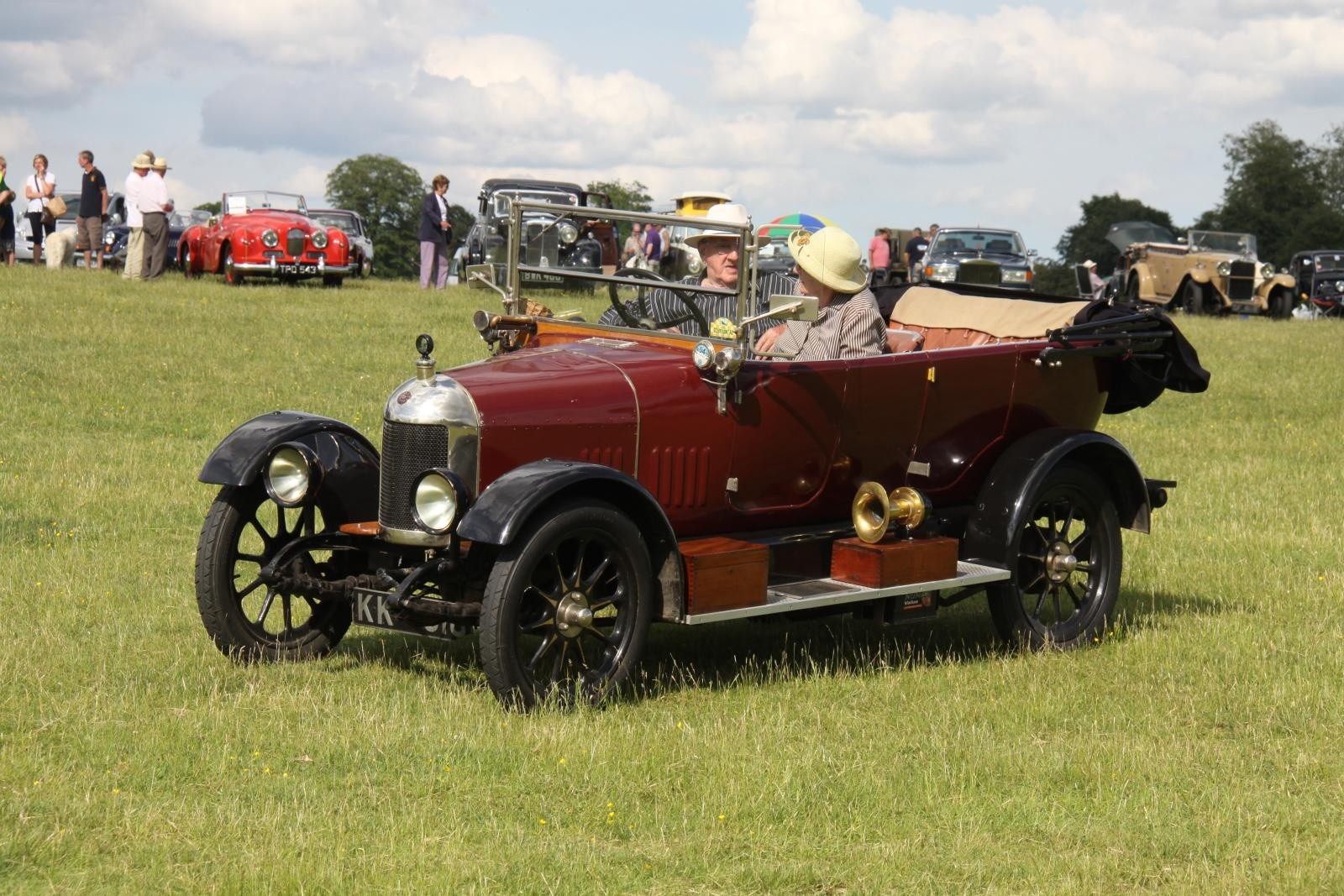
[706,240,735,254]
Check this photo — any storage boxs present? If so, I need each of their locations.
[830,532,959,588]
[677,536,769,615]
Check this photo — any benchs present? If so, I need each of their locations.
[885,286,1092,353]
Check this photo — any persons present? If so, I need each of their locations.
[121,150,175,283]
[903,223,940,282]
[597,203,799,361]
[24,154,58,266]
[417,175,452,293]
[623,222,671,291]
[771,226,886,362]
[75,151,107,271]
[1082,260,1113,301]
[0,156,17,268]
[868,228,892,273]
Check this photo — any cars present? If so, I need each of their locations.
[455,175,621,296]
[659,226,1038,292]
[1104,219,1296,321]
[14,190,373,289]
[1290,250,1344,317]
[195,190,1211,715]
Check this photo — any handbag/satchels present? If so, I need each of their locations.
[40,206,56,229]
[47,193,67,218]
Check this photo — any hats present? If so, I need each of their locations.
[684,204,772,250]
[152,158,171,170]
[132,154,155,168]
[788,225,869,294]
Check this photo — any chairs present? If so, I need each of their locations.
[938,238,966,248]
[1310,292,1344,321]
[986,240,1012,250]
[1075,265,1105,300]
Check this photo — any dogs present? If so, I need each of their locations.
[45,227,80,268]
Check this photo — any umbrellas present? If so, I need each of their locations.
[756,213,836,239]
